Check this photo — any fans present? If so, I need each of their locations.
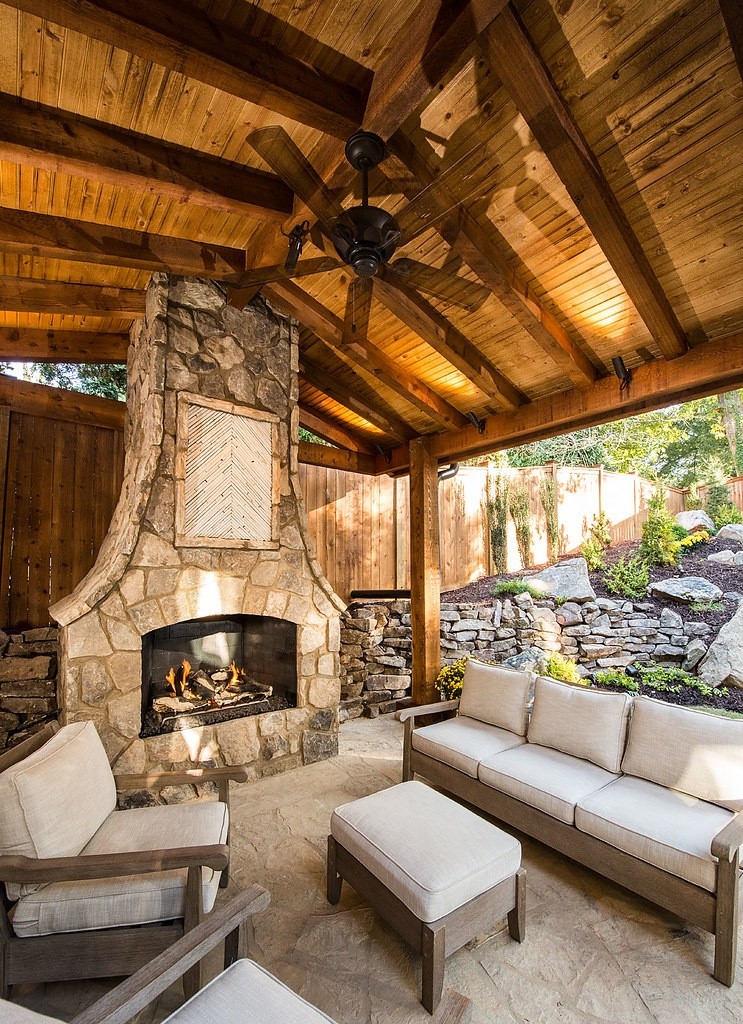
[221,122,509,348]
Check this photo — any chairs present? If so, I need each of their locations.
[0,720,340,1024]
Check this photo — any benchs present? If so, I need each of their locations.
[394,658,743,987]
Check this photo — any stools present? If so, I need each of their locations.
[328,780,528,1013]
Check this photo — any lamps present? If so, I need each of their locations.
[376,442,392,464]
[612,356,633,390]
[279,218,310,276]
[466,411,485,434]
